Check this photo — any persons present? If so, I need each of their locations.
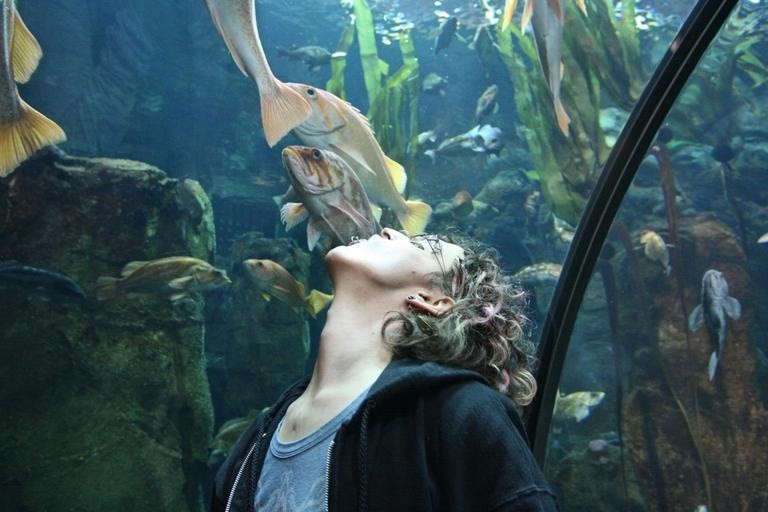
[210,229,557,512]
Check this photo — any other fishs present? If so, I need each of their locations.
[272,0,595,288]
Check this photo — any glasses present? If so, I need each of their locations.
[410,230,447,280]
